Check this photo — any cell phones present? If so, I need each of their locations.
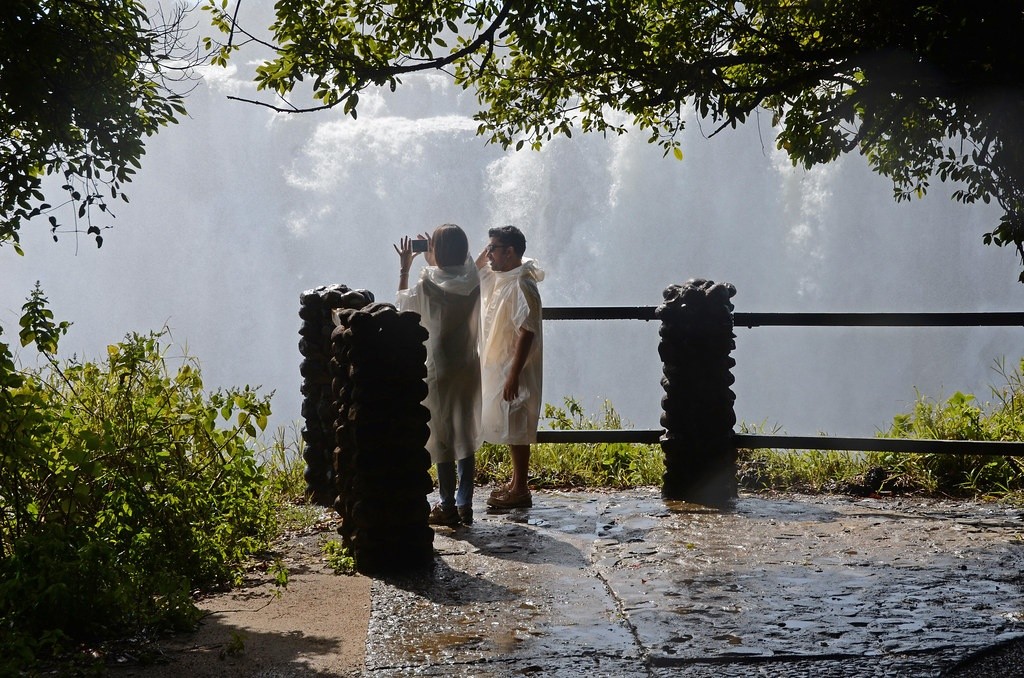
[411,239,428,253]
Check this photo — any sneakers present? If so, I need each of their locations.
[428,502,461,523]
[456,507,473,523]
[490,485,531,496]
[487,490,533,507]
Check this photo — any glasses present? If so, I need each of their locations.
[488,245,507,252]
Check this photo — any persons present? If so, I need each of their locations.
[475,226,544,508]
[393,224,484,526]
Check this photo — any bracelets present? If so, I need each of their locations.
[400,272,408,276]
